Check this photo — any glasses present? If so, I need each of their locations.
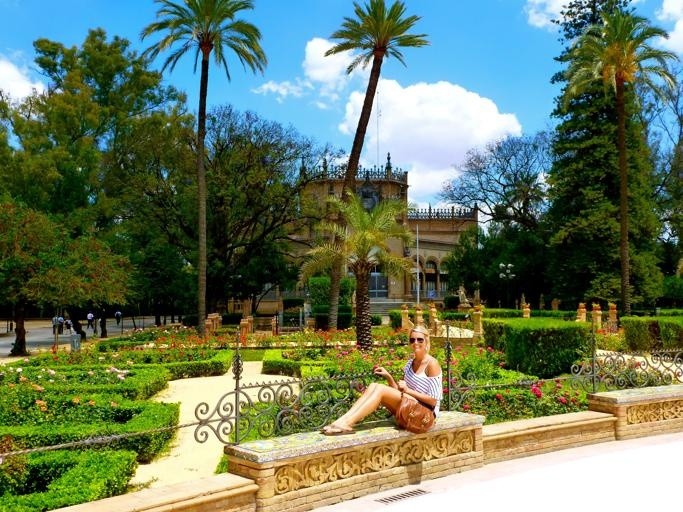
[410,337,423,344]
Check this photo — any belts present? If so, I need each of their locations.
[418,401,435,419]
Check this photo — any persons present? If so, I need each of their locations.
[65,317,72,335]
[113,309,121,325]
[85,311,94,329]
[51,314,57,334]
[56,314,64,334]
[320,325,443,438]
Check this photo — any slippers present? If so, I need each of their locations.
[319,424,333,433]
[325,426,355,436]
